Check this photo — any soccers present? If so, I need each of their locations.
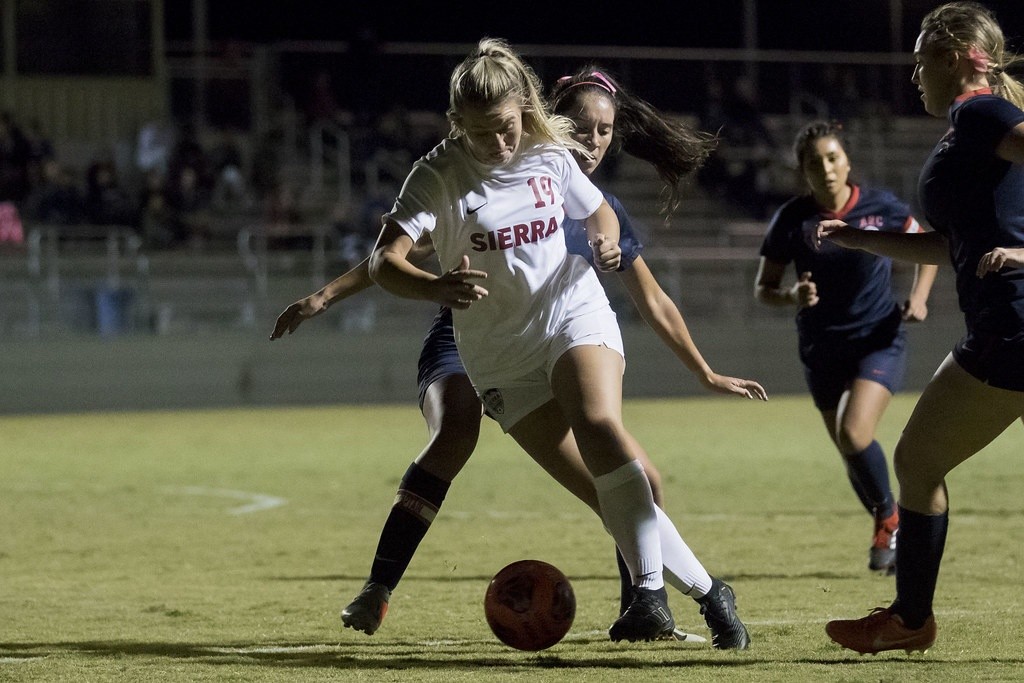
[484,560,578,652]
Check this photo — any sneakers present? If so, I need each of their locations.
[868,503,900,572]
[700,579,751,651]
[608,592,675,643]
[824,607,938,654]
[339,581,392,637]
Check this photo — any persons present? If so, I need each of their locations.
[269,66,767,641]
[750,121,937,579]
[370,38,751,652]
[826,3,1024,656]
[0,81,889,253]
